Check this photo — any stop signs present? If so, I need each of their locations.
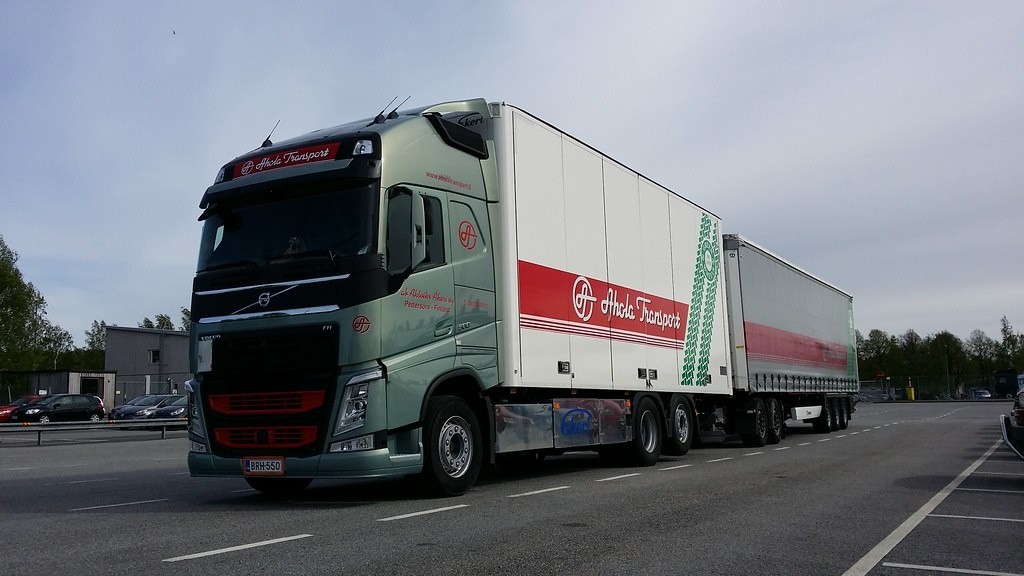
[876,371,884,380]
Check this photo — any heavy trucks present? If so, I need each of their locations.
[181,94,863,497]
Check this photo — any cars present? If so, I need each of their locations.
[976,391,992,399]
[8,393,105,423]
[0,394,46,422]
[107,393,188,429]
